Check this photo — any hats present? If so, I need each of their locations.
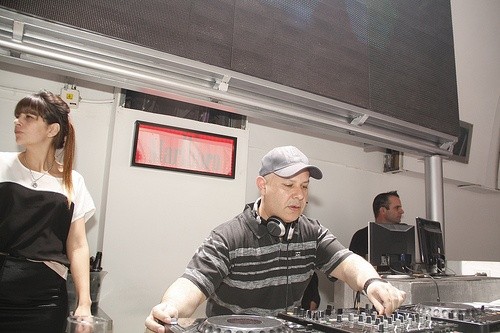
[259,145,323,180]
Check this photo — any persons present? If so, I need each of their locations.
[301,272,320,310]
[348,191,404,260]
[145,145,408,333]
[0,90,96,333]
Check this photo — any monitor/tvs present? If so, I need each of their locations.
[367,222,415,273]
[416,217,448,275]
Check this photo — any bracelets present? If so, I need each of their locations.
[363,278,388,296]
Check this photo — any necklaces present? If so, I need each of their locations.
[24,158,54,188]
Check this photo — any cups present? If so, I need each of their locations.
[65,315,113,333]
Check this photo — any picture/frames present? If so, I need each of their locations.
[131,120,237,180]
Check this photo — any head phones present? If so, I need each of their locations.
[252,197,298,241]
